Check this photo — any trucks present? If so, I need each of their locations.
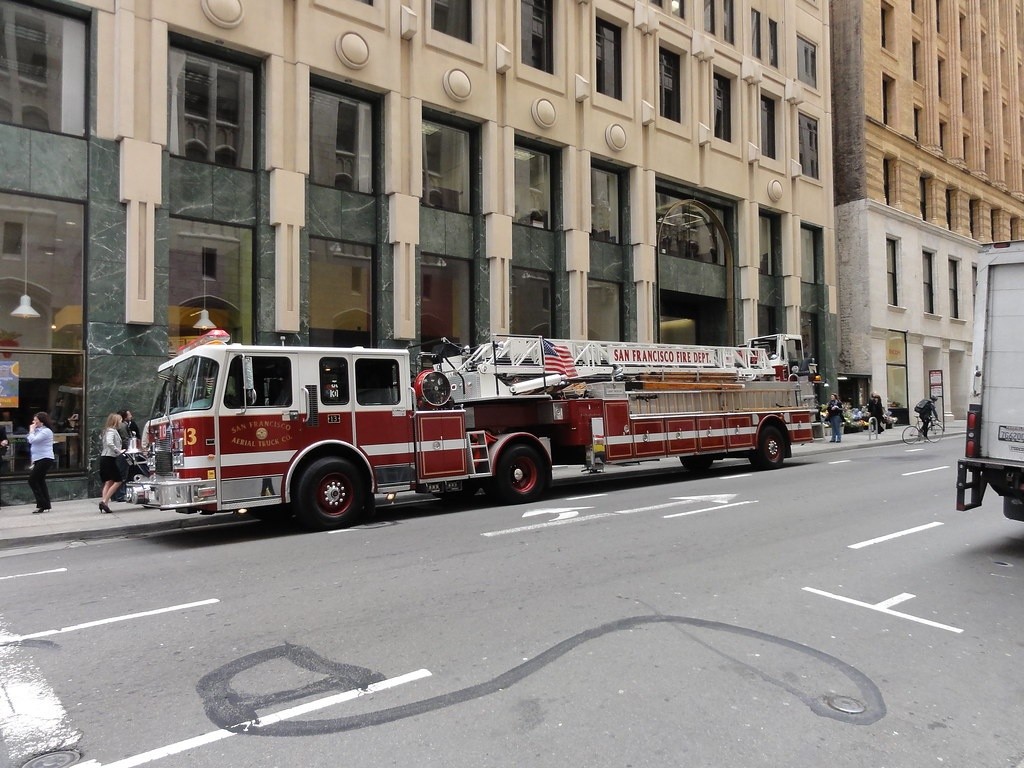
[957,240,1024,521]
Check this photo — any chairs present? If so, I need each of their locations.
[268,378,290,405]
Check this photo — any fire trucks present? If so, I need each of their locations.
[123,330,823,533]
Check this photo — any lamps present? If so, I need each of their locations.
[10,214,41,319]
[192,245,217,331]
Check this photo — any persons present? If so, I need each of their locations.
[868,393,885,434]
[99,415,123,513]
[918,396,939,442]
[111,410,141,502]
[0,426,9,506]
[801,354,814,371]
[28,413,54,513]
[828,393,843,442]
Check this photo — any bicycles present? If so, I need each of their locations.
[902,415,943,444]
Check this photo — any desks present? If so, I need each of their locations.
[8,432,79,472]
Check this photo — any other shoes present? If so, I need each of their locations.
[111,494,126,502]
[923,432,927,437]
[836,441,841,442]
[830,440,835,442]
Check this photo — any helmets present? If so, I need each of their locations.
[931,395,938,400]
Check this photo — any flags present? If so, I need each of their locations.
[543,340,578,377]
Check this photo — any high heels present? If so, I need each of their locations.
[32,506,50,514]
[100,502,112,513]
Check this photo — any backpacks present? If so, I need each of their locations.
[915,400,932,412]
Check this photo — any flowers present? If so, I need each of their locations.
[820,402,869,427]
